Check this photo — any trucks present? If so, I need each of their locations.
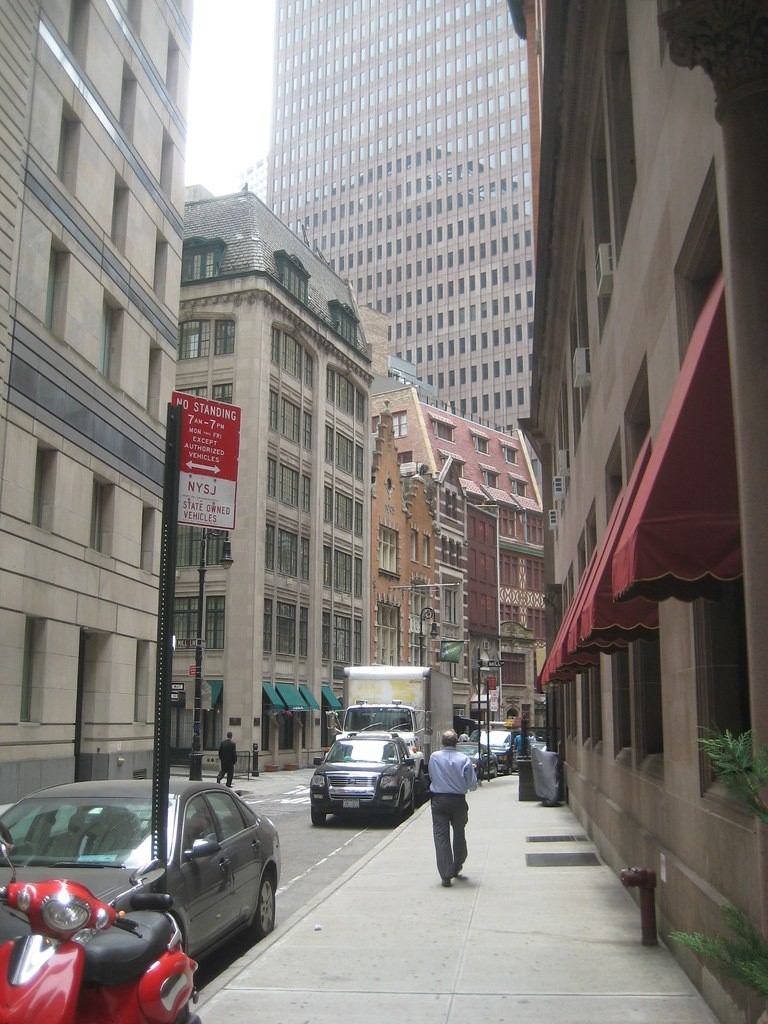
[326,666,453,806]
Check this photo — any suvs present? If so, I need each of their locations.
[309,731,416,825]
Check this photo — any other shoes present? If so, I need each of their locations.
[217,778,220,783]
[226,785,232,788]
[441,877,451,888]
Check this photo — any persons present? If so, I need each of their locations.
[216,732,238,789]
[427,730,480,888]
[505,726,537,774]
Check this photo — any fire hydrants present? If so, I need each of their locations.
[621,865,658,945]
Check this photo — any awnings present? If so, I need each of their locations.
[610,267,743,607]
[263,680,285,711]
[201,678,223,709]
[320,684,341,709]
[534,427,659,699]
[299,684,321,710]
[276,681,310,712]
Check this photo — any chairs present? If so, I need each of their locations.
[17,805,142,860]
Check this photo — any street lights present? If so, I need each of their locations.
[420,607,438,666]
[189,530,234,780]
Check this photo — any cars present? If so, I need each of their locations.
[480,729,521,775]
[455,742,498,781]
[0,778,281,961]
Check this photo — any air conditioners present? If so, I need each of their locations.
[556,448,570,477]
[572,347,591,388]
[551,476,564,502]
[548,509,558,530]
[594,242,613,298]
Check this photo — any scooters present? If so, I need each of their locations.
[0,821,201,1024]
